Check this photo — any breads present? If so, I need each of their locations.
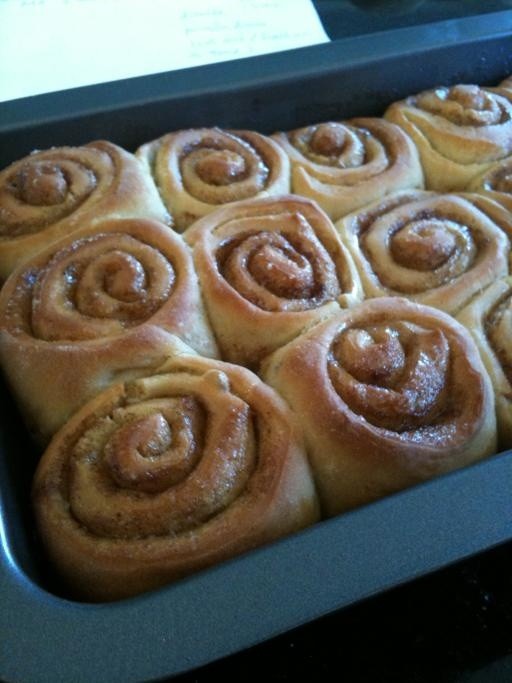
[0,76,512,599]
